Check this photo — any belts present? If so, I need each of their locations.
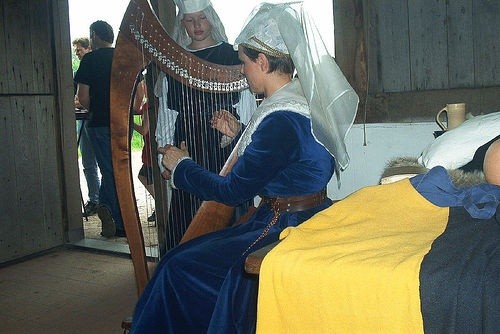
[263,186,327,212]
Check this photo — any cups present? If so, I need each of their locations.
[437,102,466,132]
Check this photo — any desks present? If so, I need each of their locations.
[244,173,499,333]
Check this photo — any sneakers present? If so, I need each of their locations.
[146,211,156,222]
[114,222,127,238]
[98,202,116,237]
[82,201,102,216]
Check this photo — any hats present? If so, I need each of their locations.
[237,1,290,58]
[174,0,210,13]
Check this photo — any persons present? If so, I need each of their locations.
[131,3,339,334]
[128,74,157,226]
[73,37,103,217]
[155,0,257,255]
[76,18,131,240]
